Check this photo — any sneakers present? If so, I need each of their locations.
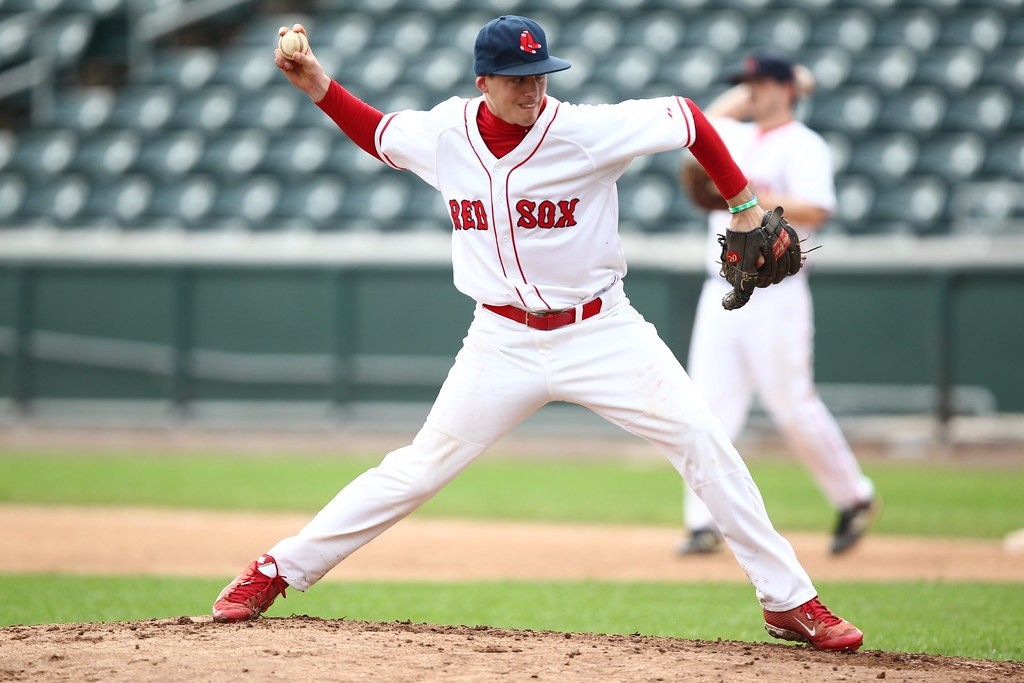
[212,554,290,622]
[687,532,718,552]
[831,494,881,554]
[763,596,864,652]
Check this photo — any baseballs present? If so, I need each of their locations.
[278,30,309,60]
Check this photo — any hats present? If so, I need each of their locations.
[709,45,793,86]
[474,15,572,77]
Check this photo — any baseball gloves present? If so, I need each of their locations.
[681,158,733,209]
[714,206,823,310]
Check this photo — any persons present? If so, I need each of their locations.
[212,15,864,653]
[675,55,878,558]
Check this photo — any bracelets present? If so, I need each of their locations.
[729,196,760,213]
